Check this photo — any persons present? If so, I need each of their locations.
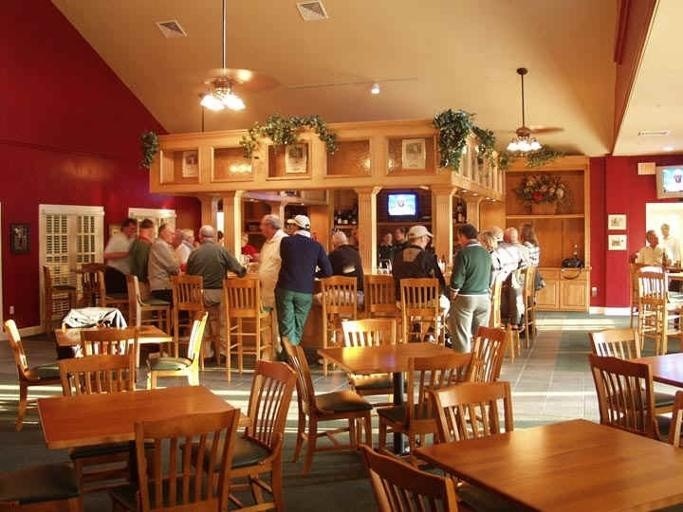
[658,223,682,267]
[256,214,289,362]
[392,225,445,344]
[479,222,541,338]
[391,226,408,258]
[315,231,364,342]
[637,229,667,263]
[379,233,393,267]
[446,225,494,383]
[104,218,255,362]
[273,214,333,362]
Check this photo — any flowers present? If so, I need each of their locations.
[511,173,576,209]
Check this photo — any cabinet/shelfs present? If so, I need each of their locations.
[505,169,590,312]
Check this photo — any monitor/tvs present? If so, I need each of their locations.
[655,165,683,200]
[386,192,420,222]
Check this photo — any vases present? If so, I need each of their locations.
[530,200,556,215]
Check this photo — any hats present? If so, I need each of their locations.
[408,225,434,240]
[286,214,310,230]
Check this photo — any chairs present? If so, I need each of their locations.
[588,328,676,418]
[636,272,683,357]
[589,353,682,442]
[1,262,513,511]
[501,267,539,350]
[629,251,667,328]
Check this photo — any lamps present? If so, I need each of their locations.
[201,0,254,112]
[506,68,542,151]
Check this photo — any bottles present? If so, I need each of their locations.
[457,199,463,213]
[439,259,443,273]
[337,209,357,224]
[442,254,446,273]
[458,208,462,222]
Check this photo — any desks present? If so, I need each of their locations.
[415,418,682,512]
[627,353,683,388]
